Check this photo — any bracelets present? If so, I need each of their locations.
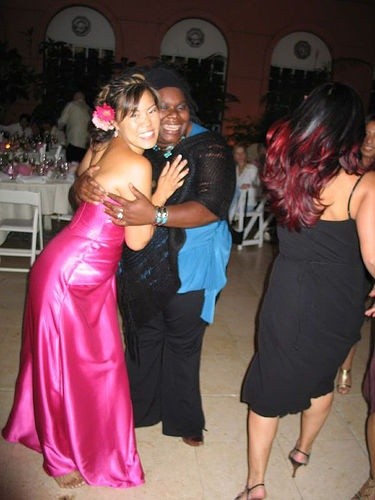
[158,205,169,226]
[153,205,162,226]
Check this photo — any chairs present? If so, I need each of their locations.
[0,185,276,273]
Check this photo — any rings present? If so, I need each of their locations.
[120,208,124,213]
[118,212,123,219]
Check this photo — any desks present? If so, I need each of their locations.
[0,177,76,246]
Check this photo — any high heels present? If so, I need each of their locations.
[288,448,310,478]
[234,484,265,500]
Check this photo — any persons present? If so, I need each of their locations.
[225,144,261,252]
[0,114,34,143]
[40,120,66,146]
[57,90,93,171]
[236,79,375,500]
[69,68,236,446]
[1,72,189,490]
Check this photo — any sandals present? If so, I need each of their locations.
[337,368,351,395]
